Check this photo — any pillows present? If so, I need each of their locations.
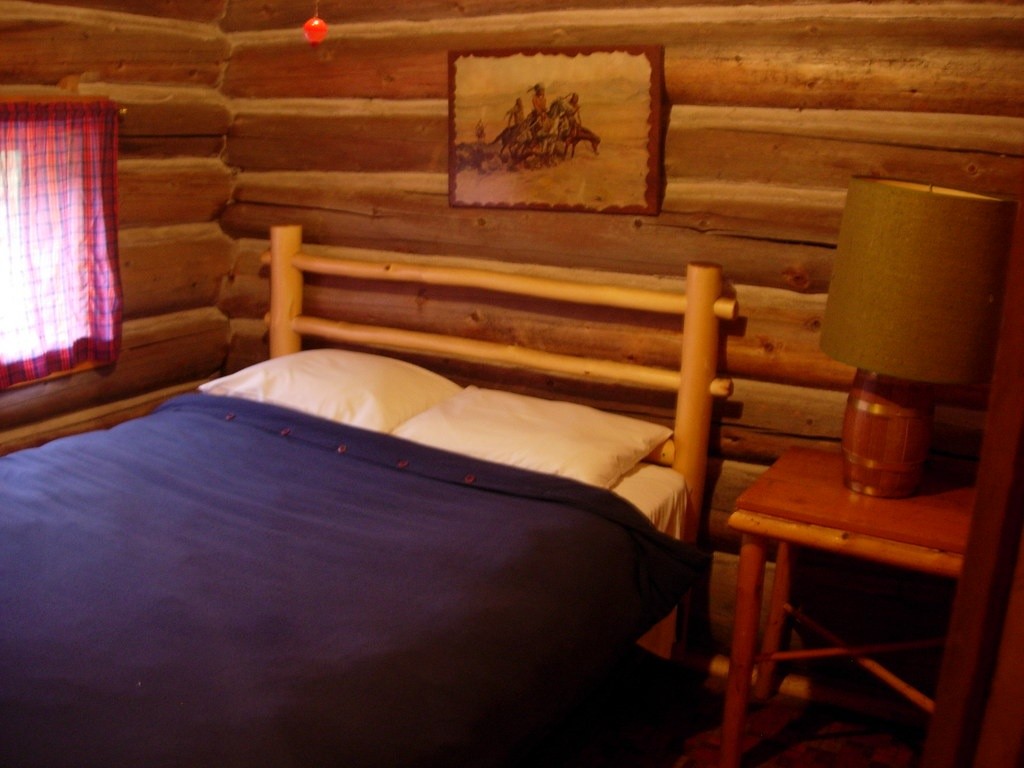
[394,383,670,490]
[196,347,463,436]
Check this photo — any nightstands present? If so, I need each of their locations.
[717,444,978,768]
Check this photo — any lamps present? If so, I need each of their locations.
[819,175,1016,502]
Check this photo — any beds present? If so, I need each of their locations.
[0,227,743,768]
